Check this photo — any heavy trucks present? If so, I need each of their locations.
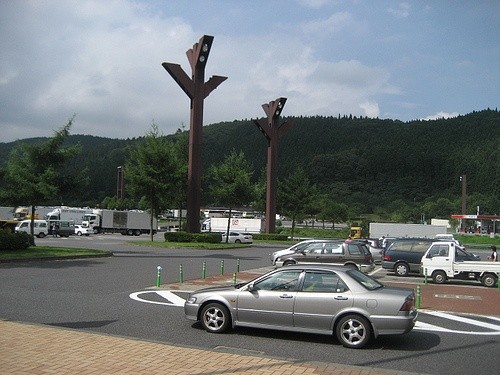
[82,209,158,236]
[349,223,448,240]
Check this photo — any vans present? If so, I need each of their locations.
[46,220,76,237]
[14,219,48,238]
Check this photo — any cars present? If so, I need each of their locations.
[75,224,94,236]
[183,264,419,349]
[270,234,481,275]
[221,231,253,244]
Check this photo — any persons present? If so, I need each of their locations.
[382,236,387,248]
[487,246,498,262]
[52,222,57,237]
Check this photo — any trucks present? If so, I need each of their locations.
[0,215,39,234]
[420,242,498,289]
[201,218,261,235]
[45,208,92,224]
[13,208,45,220]
